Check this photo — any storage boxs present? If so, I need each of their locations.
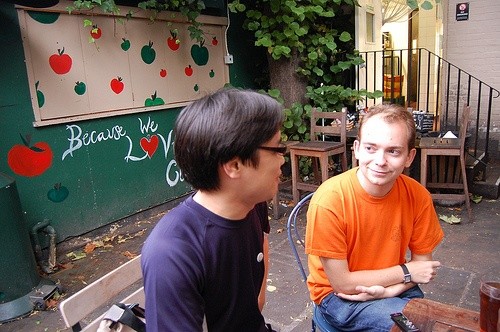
[383,74,404,99]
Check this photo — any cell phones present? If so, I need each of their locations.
[390,313,419,332]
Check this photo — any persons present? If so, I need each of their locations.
[138,87,288,332]
[305,103,444,332]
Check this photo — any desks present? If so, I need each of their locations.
[390,298,480,332]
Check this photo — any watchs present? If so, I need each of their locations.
[397,264,411,285]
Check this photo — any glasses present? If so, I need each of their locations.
[248,140,288,158]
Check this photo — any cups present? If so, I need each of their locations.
[479,272,500,332]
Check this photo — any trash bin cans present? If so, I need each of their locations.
[0,175,58,324]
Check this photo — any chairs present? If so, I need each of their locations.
[289,107,347,205]
[273,141,300,221]
[287,192,316,332]
[419,103,474,223]
[59,254,145,332]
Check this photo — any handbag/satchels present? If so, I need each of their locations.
[97,302,146,332]
